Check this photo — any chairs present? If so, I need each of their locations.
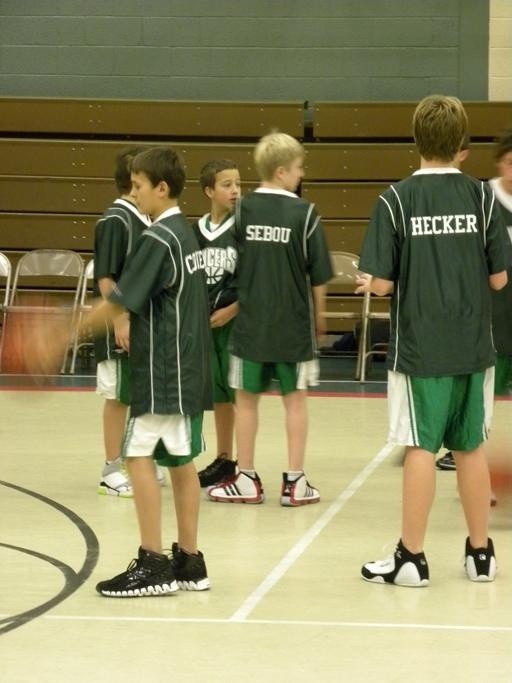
[0,249,85,375]
[318,250,366,383]
[69,255,97,374]
[359,270,392,382]
[0,250,12,335]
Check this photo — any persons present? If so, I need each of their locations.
[437,130,512,473]
[18,144,211,597]
[93,144,173,499]
[191,158,246,488]
[207,130,339,505]
[353,91,511,589]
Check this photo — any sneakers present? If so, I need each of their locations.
[170,544,211,592]
[361,539,430,587]
[280,470,321,505]
[208,473,264,505]
[464,535,498,582]
[155,462,172,486]
[198,458,236,487]
[98,460,135,497]
[96,547,179,598]
[436,451,458,471]
